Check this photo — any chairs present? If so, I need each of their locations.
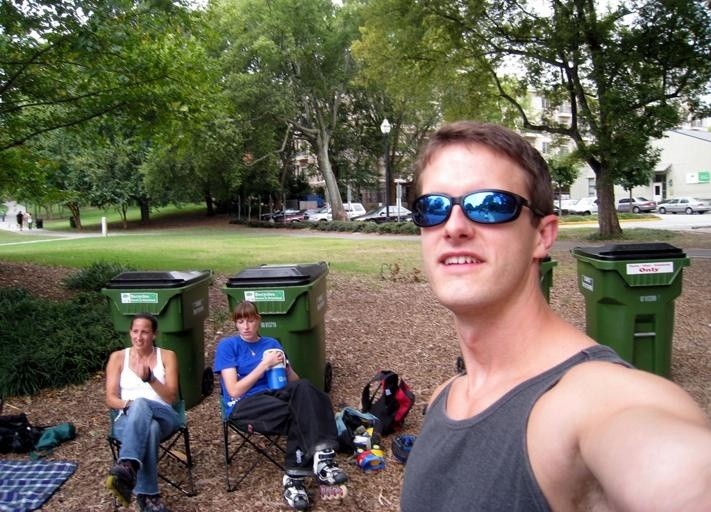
[219,342,296,493]
[106,367,195,498]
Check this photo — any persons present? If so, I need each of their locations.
[15,210,25,231]
[401,120,709,511]
[212,300,351,511]
[104,312,186,511]
[26,211,33,230]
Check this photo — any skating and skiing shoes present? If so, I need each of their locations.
[283,474,310,512]
[135,494,173,512]
[106,460,137,509]
[313,449,348,501]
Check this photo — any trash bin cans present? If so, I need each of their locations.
[101,270,214,411]
[219,261,332,393]
[456,256,558,376]
[36,219,42,228]
[569,242,691,379]
[70,217,77,228]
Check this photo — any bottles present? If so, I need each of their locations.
[264,348,290,390]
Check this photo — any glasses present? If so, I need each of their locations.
[411,190,545,227]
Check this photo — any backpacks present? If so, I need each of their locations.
[362,371,415,437]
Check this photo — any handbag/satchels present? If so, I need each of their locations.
[35,422,75,452]
[335,407,381,454]
[0,413,43,455]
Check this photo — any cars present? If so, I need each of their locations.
[395,213,414,222]
[260,209,281,220]
[282,208,321,224]
[658,197,711,215]
[265,211,276,222]
[350,206,412,224]
[273,210,300,221]
[553,197,599,215]
[618,197,656,213]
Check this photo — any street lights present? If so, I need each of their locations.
[380,118,394,223]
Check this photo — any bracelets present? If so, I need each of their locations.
[139,363,155,384]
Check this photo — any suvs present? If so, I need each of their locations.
[304,203,367,224]
